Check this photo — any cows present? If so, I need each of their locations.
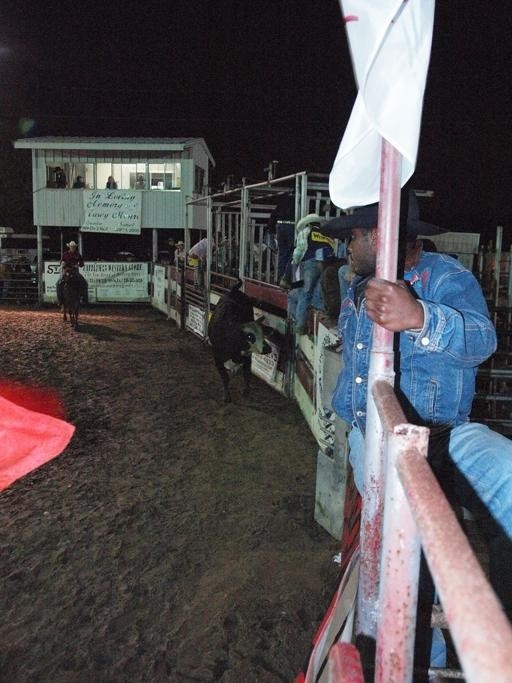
[207,271,273,406]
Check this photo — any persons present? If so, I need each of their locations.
[174,240,185,265]
[268,185,300,289]
[53,167,66,188]
[134,173,147,190]
[189,231,222,290]
[72,176,86,188]
[106,176,117,189]
[57,241,88,307]
[320,206,512,539]
[280,213,348,335]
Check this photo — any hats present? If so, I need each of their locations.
[321,190,447,239]
[66,241,80,247]
[297,214,321,232]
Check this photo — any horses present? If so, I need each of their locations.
[59,266,82,332]
